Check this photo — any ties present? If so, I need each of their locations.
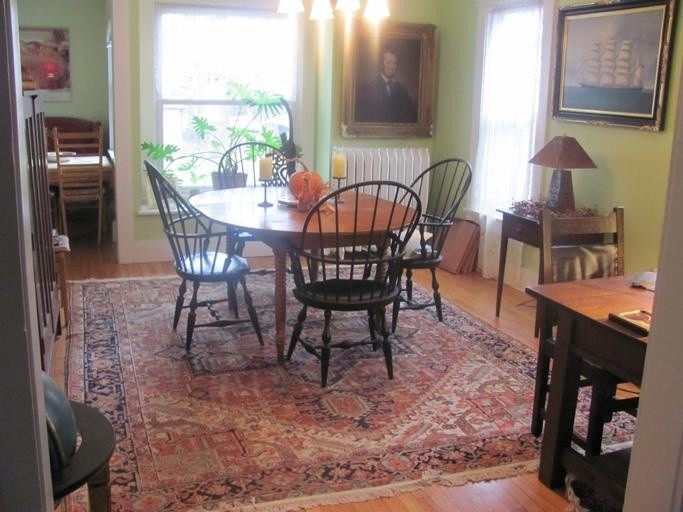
[387,79,394,93]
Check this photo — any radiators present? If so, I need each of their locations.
[328,144,431,263]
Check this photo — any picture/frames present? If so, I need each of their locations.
[143,176,180,209]
[552,0,681,134]
[338,13,438,140]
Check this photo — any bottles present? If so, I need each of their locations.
[297,172,315,212]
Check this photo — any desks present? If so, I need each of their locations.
[493,204,618,338]
[184,180,420,366]
[46,153,114,188]
[52,400,117,512]
[523,269,655,489]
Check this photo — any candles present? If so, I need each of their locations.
[331,153,347,177]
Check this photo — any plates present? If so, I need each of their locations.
[277,198,298,208]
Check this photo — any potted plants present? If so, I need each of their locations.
[139,86,279,190]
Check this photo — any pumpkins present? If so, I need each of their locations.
[288,169,323,202]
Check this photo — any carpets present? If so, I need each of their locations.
[57,265,641,511]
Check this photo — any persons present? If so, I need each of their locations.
[357,43,417,123]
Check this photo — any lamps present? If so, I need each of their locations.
[529,133,598,210]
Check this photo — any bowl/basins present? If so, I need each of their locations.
[47,151,76,163]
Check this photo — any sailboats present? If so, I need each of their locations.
[578,31,645,93]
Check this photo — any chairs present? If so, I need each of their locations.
[215,142,306,314]
[50,123,109,249]
[529,207,643,463]
[138,159,265,352]
[278,177,424,384]
[384,157,471,333]
